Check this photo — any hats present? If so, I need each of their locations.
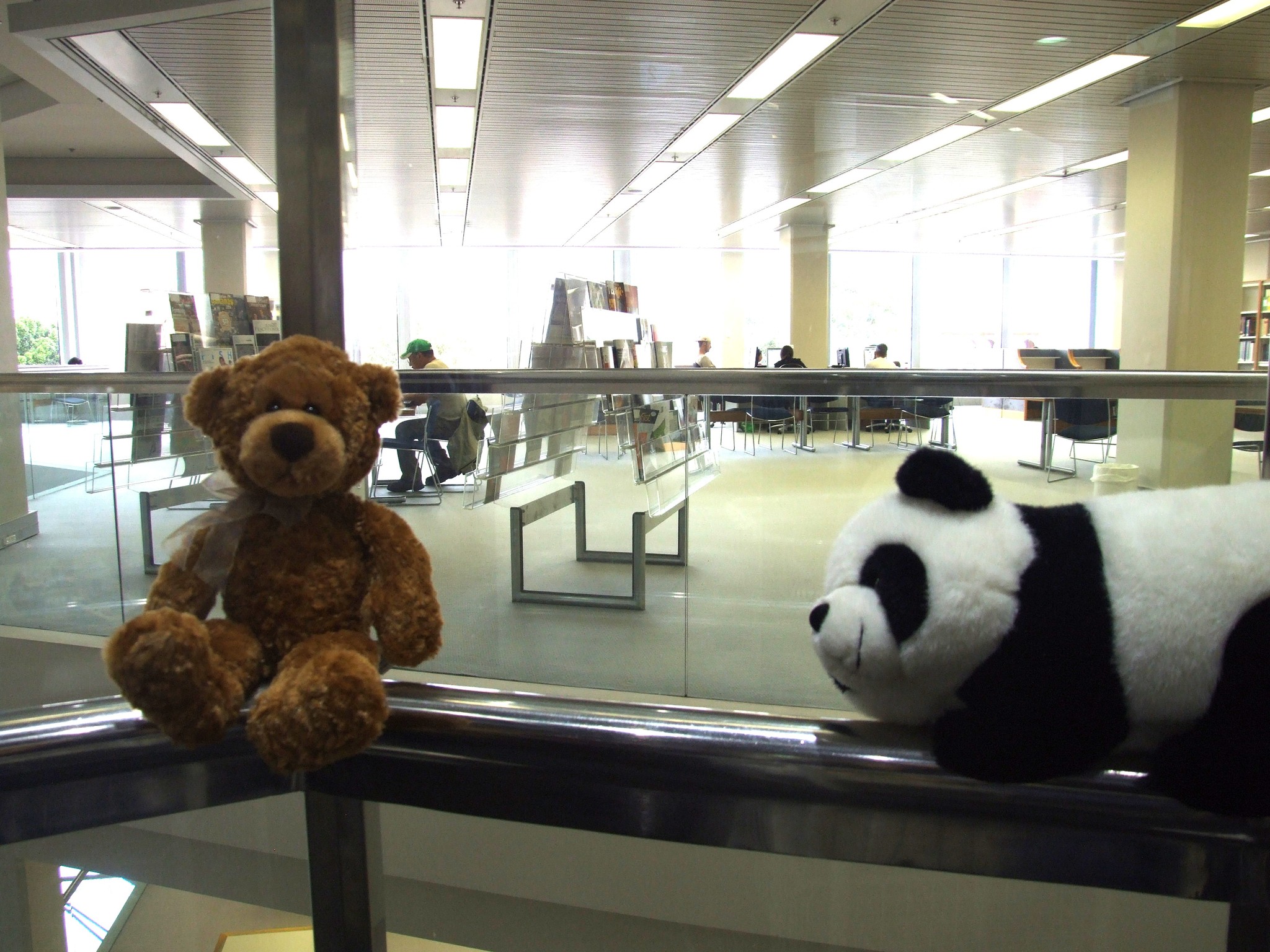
[401,339,433,359]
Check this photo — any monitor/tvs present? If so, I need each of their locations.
[767,348,783,367]
[863,346,878,369]
[836,347,850,367]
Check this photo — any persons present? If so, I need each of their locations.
[758,345,807,431]
[259,310,268,320]
[219,357,228,367]
[254,314,260,320]
[387,338,468,493]
[695,337,715,367]
[68,357,83,365]
[865,344,900,429]
[229,360,232,365]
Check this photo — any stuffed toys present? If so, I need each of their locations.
[102,334,444,778]
[808,444,1270,818]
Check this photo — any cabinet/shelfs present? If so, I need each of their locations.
[463,272,725,611]
[83,345,267,509]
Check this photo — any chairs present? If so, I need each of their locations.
[370,400,443,506]
[411,395,482,493]
[806,397,849,449]
[49,393,96,424]
[720,396,797,456]
[1047,398,1117,482]
[859,397,957,452]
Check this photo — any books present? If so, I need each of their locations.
[252,320,282,353]
[124,323,163,373]
[169,333,199,371]
[169,293,201,335]
[233,335,257,361]
[483,278,701,504]
[199,347,233,372]
[130,393,165,461]
[209,292,273,346]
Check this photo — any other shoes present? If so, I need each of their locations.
[426,464,458,485]
[387,475,425,492]
